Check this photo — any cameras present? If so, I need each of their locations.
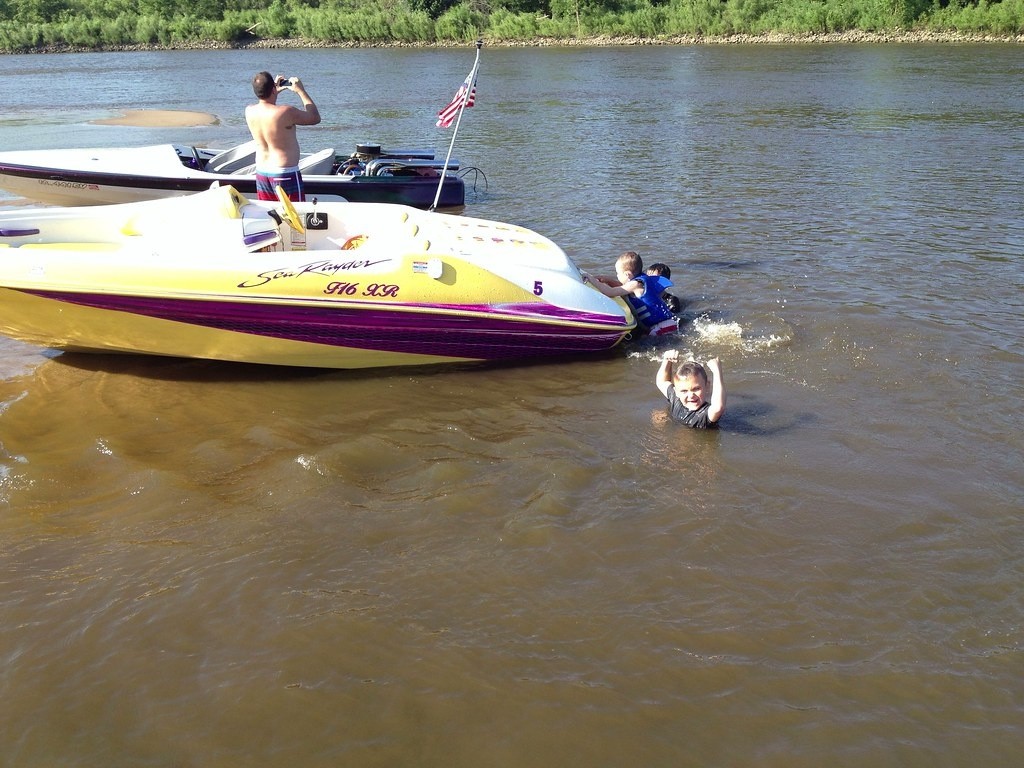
[279,79,292,87]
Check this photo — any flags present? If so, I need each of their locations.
[436,58,480,128]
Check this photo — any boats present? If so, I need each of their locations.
[0,142,467,218]
[0,183,638,375]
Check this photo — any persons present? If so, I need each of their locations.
[583,252,680,337]
[656,350,725,430]
[244,71,320,202]
